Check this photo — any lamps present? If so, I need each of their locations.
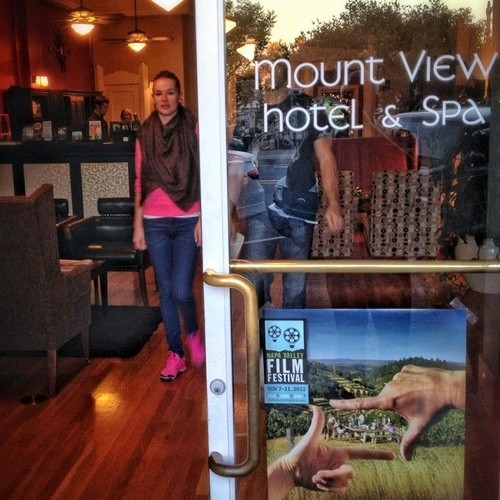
[126,0,150,52]
[68,0,95,35]
[152,0,183,12]
[225,19,236,33]
[236,44,255,61]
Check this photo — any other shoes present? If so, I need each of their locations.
[254,272,275,312]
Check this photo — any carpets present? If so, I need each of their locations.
[22,304,162,359]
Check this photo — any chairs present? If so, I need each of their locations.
[0,183,93,396]
[54,197,158,307]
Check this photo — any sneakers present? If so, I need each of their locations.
[159,330,205,383]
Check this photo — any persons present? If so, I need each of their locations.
[82,96,110,143]
[228,41,344,309]
[120,109,132,123]
[133,70,205,381]
[267,364,466,500]
